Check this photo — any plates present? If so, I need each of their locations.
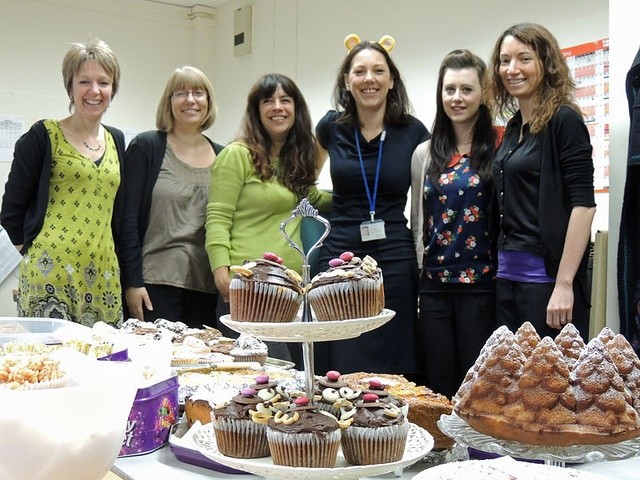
[218,308,396,342]
[193,421,435,480]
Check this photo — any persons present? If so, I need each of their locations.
[124,65,227,326]
[1,40,126,324]
[490,23,596,343]
[205,74,332,369]
[311,33,426,385]
[408,49,498,394]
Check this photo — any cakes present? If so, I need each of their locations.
[342,372,453,451]
[305,251,390,322]
[266,396,341,468]
[341,393,409,465]
[452,321,640,447]
[210,388,272,458]
[114,318,268,376]
[228,253,305,323]
[212,370,407,410]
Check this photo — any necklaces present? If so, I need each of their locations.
[73,117,102,152]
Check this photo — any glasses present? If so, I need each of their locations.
[170,89,207,99]
